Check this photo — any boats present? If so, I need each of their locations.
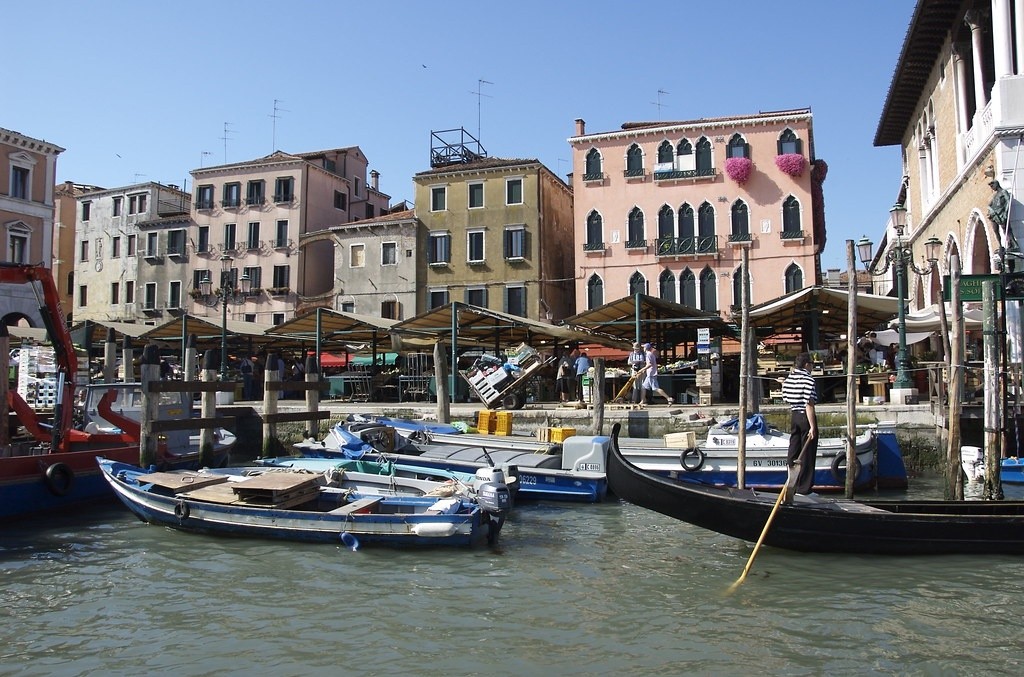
[0,313,239,526]
[606,422,1024,556]
[292,410,911,506]
[95,455,512,551]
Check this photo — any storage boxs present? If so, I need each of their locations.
[537,426,552,442]
[863,396,884,406]
[663,431,695,448]
[551,428,576,443]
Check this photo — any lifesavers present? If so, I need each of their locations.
[831,452,862,484]
[680,447,704,471]
[45,462,73,497]
[406,430,426,445]
[174,502,190,521]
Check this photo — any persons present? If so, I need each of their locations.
[395,362,407,372]
[782,351,819,496]
[240,354,265,401]
[893,175,910,206]
[277,352,285,400]
[161,360,178,379]
[986,179,1019,251]
[73,389,88,409]
[199,355,205,370]
[500,353,523,379]
[458,356,473,369]
[977,343,985,361]
[826,333,901,375]
[626,341,675,408]
[558,349,591,404]
[292,356,305,375]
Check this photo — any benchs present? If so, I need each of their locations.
[328,496,384,514]
[421,499,460,514]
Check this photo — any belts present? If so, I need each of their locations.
[794,411,805,413]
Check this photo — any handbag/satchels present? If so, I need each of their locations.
[241,360,251,373]
[300,371,305,380]
[562,366,570,376]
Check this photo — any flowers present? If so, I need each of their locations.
[774,153,805,176]
[724,156,754,184]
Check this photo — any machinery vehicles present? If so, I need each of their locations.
[0,259,78,453]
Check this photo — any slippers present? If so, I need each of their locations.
[668,398,674,407]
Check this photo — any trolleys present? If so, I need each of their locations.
[458,356,559,412]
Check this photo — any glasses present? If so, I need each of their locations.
[807,361,814,365]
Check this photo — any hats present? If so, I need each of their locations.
[641,343,651,348]
[633,343,640,350]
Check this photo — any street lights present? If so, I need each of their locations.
[198,248,251,383]
[854,199,943,388]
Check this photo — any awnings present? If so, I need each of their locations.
[352,352,398,366]
[307,351,355,367]
